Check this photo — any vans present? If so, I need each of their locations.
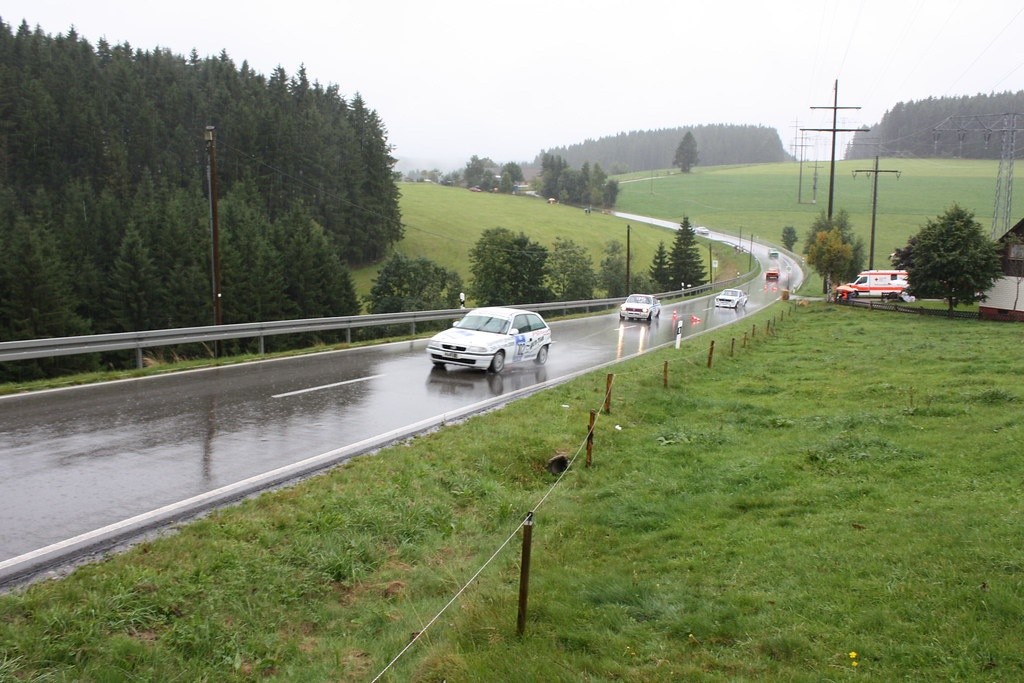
[835,269,916,299]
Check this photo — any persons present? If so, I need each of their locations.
[838,291,852,303]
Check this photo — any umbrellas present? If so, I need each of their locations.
[835,285,853,293]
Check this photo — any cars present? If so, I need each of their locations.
[715,289,748,309]
[696,227,709,235]
[765,268,780,279]
[425,307,552,374]
[768,248,779,258]
[619,294,662,321]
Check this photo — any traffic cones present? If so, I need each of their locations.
[672,308,678,320]
[689,315,701,322]
